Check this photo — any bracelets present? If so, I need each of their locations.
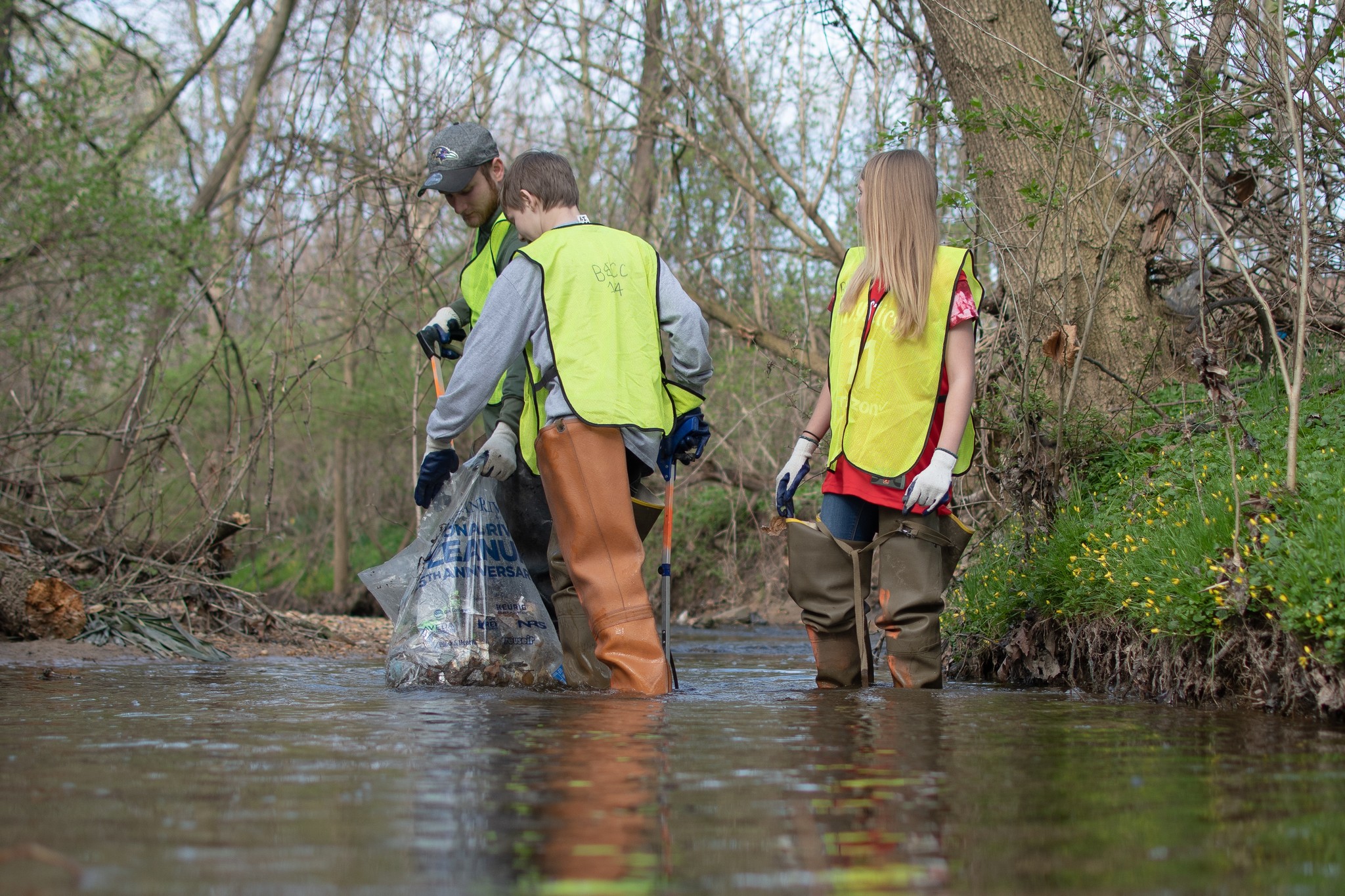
[803,431,820,442]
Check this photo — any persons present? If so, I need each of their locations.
[774,148,986,689]
[416,122,559,638]
[412,150,714,693]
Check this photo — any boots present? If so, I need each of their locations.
[783,518,872,689]
[874,502,974,687]
[533,417,672,697]
[545,481,665,692]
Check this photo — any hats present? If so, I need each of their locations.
[417,122,499,197]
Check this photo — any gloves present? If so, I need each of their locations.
[414,435,460,509]
[473,422,519,482]
[420,307,462,360]
[664,407,710,466]
[775,436,819,520]
[901,448,958,517]
